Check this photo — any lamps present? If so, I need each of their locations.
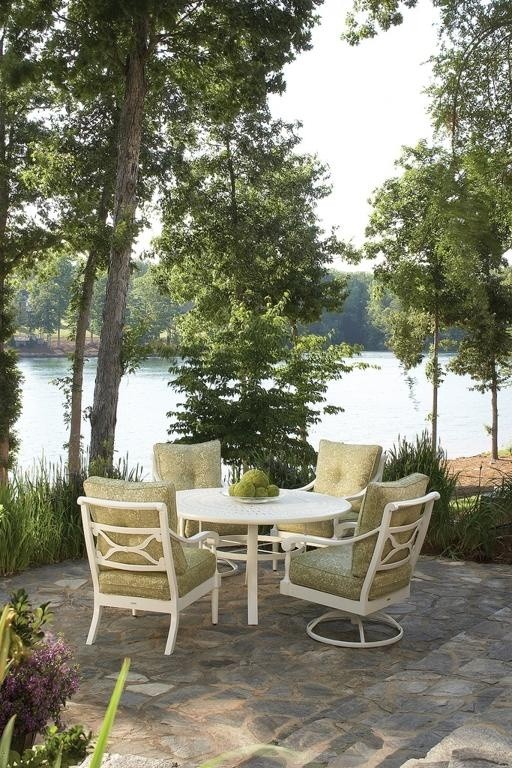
[77,476,220,657]
[269,438,389,573]
[279,471,441,649]
[153,437,249,580]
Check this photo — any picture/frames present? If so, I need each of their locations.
[173,485,354,626]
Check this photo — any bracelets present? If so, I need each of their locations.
[218,487,284,504]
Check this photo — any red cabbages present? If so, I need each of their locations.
[228,470,279,497]
